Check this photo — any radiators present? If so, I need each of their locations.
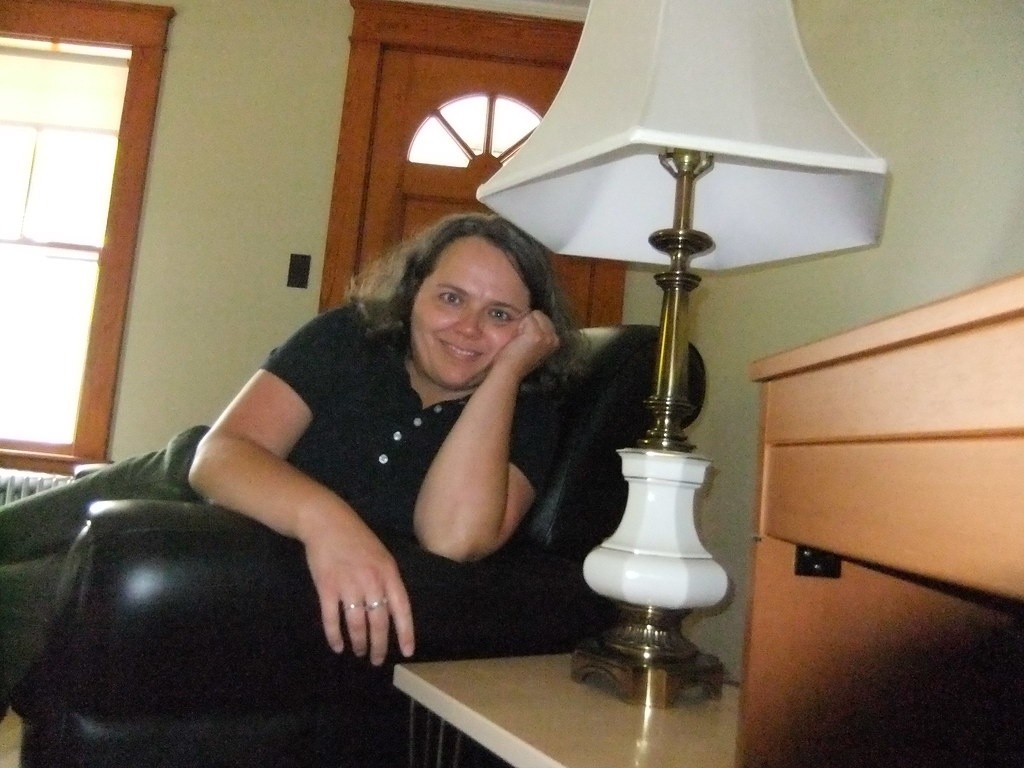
[0,468,75,507]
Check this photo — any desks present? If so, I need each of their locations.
[392,655,737,768]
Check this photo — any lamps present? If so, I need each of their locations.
[475,0,893,712]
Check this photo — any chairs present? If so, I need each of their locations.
[22,325,708,768]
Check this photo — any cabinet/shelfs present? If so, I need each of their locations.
[734,269,1024,768]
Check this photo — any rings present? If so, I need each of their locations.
[342,603,363,610]
[365,599,387,611]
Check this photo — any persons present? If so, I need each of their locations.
[0,212,579,723]
